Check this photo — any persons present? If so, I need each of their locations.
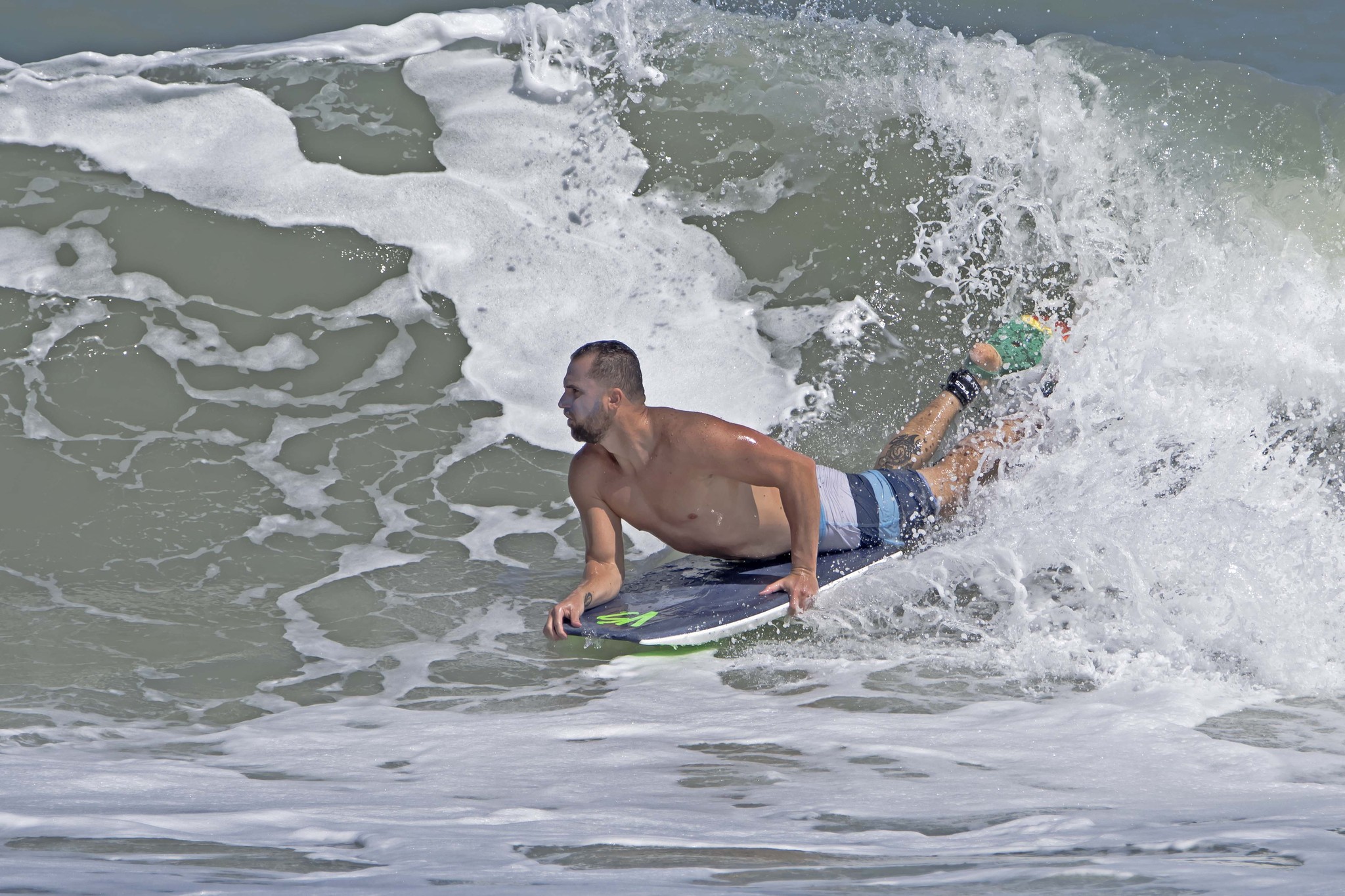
[541,315,1065,640]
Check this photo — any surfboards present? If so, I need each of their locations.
[557,542,927,648]
